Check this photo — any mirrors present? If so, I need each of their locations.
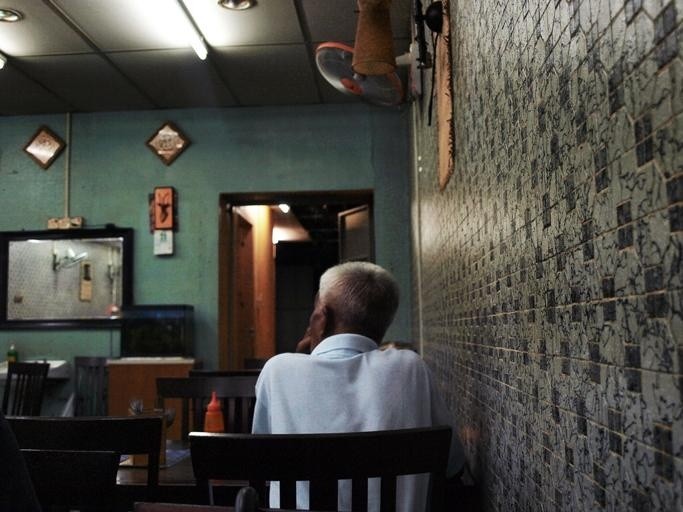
[0,227,135,330]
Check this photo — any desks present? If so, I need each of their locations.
[112,447,247,505]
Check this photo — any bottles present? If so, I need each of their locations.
[7,340,18,363]
[205,389,224,433]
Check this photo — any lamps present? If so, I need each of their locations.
[351,0,394,74]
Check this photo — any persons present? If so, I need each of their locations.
[250,260,433,511]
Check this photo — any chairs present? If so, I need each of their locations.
[2,447,122,512]
[3,358,263,427]
[186,426,459,512]
[5,414,158,502]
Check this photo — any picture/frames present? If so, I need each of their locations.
[145,121,190,165]
[23,125,67,170]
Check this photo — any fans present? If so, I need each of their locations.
[314,41,422,110]
[53,247,87,271]
